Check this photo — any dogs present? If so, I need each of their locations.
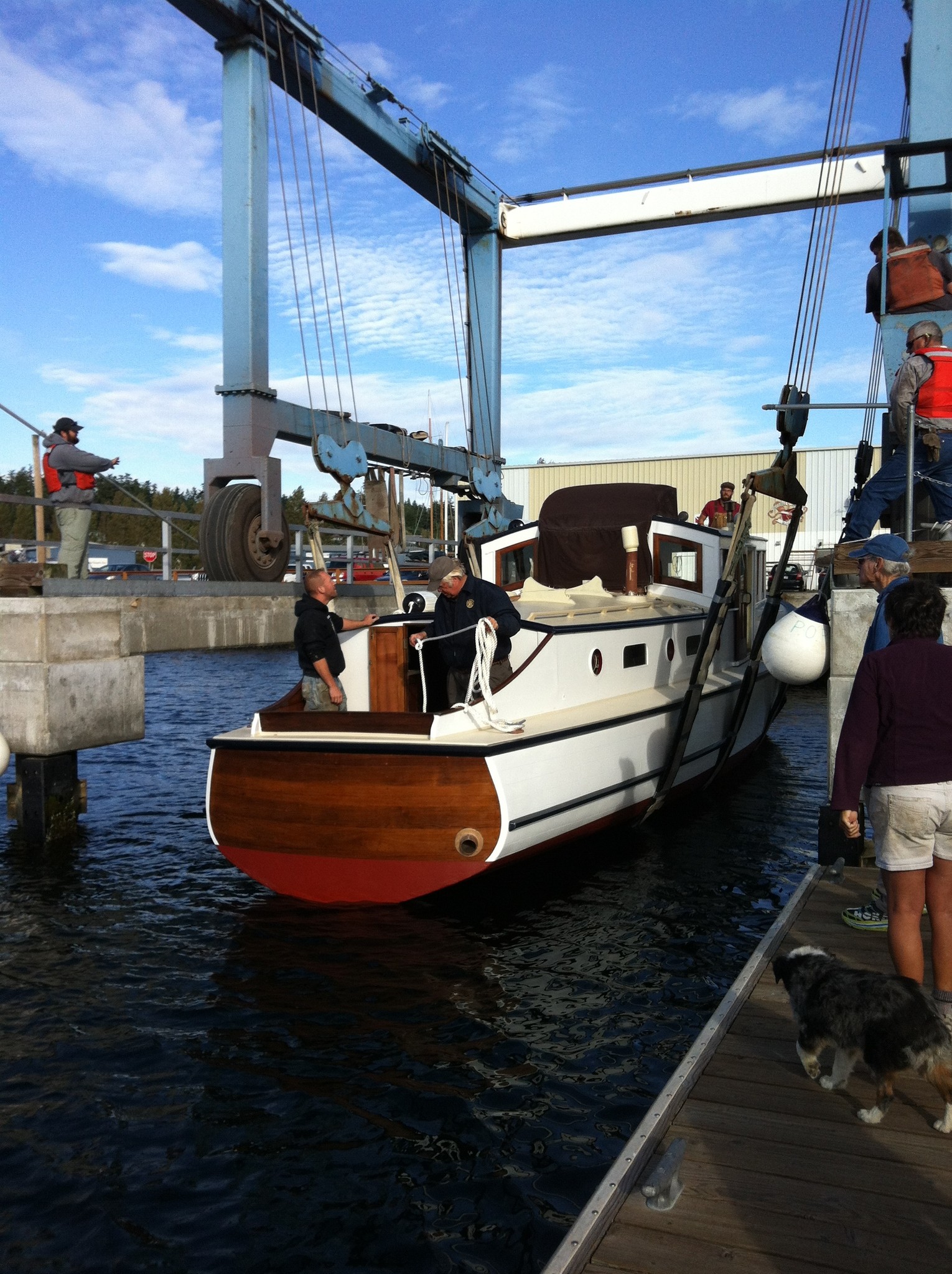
[773,945,952,1135]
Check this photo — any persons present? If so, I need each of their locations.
[42,417,120,579]
[866,226,952,323]
[294,568,380,711]
[840,321,952,544]
[832,579,952,1029]
[841,533,943,930]
[698,482,740,571]
[409,556,522,703]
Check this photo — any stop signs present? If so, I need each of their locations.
[143,551,158,562]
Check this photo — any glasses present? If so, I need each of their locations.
[906,334,932,349]
[858,557,875,564]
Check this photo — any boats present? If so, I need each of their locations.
[204,474,799,915]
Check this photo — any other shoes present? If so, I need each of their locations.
[871,888,928,915]
[841,903,889,932]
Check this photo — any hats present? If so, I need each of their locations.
[53,417,84,431]
[426,556,459,591]
[848,534,910,562]
[720,482,735,489]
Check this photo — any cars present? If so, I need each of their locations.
[328,555,387,583]
[10,540,193,581]
[373,559,431,581]
[767,562,808,592]
